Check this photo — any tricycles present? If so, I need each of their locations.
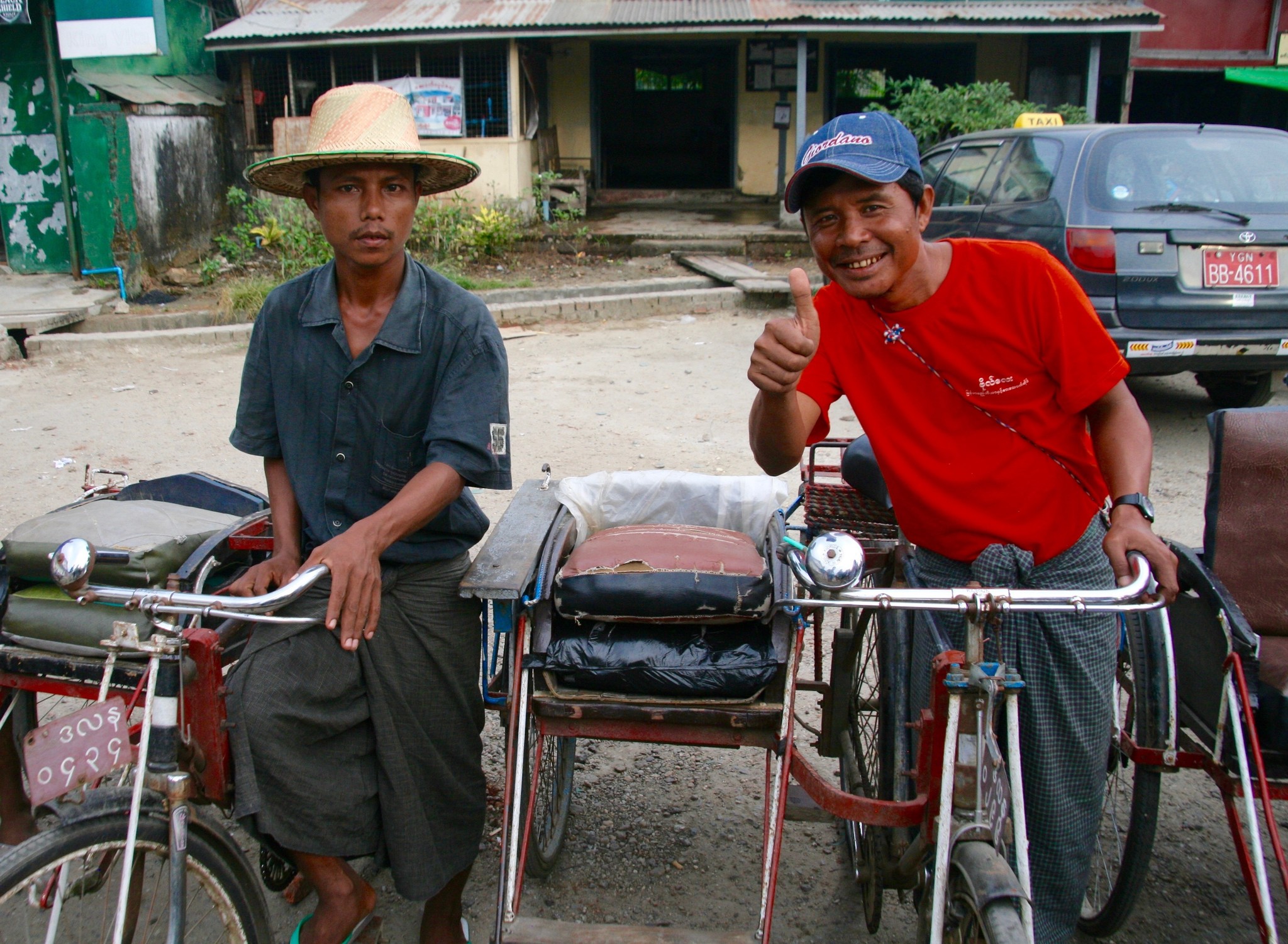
[0,400,1288,943]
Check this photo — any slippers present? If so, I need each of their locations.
[290,908,375,944]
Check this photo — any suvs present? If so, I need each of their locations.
[922,111,1288,406]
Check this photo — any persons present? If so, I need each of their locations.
[744,113,1181,944]
[222,82,513,944]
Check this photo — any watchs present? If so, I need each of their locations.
[1109,492,1155,525]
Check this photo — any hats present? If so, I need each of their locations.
[784,109,924,212]
[242,83,482,197]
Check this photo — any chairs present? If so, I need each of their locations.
[5,469,268,693]
[1204,403,1288,777]
[535,123,594,221]
[530,470,788,731]
